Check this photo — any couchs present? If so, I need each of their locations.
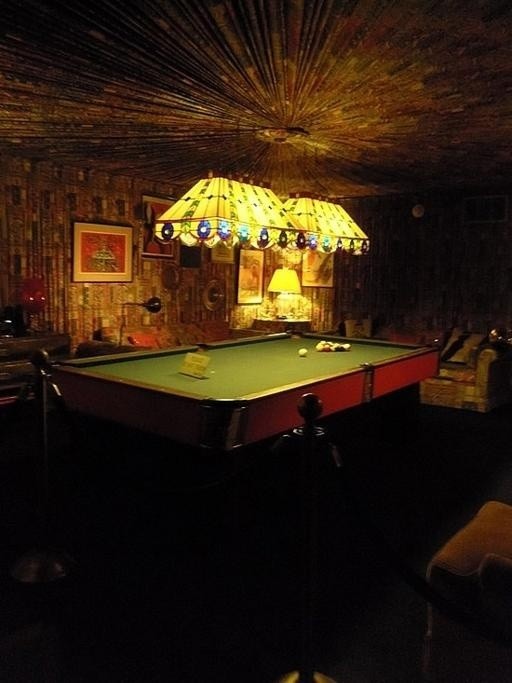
[75,318,265,357]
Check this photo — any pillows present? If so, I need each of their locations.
[438,325,487,364]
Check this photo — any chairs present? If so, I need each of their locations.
[421,498,510,681]
[417,339,509,412]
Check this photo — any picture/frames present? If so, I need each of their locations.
[68,218,133,283]
[299,250,335,288]
[233,246,265,304]
[140,191,178,259]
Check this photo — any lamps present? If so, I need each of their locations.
[149,171,371,257]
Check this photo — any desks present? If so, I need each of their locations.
[32,326,438,469]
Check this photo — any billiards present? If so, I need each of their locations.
[316,340,351,352]
[298,349,308,356]
